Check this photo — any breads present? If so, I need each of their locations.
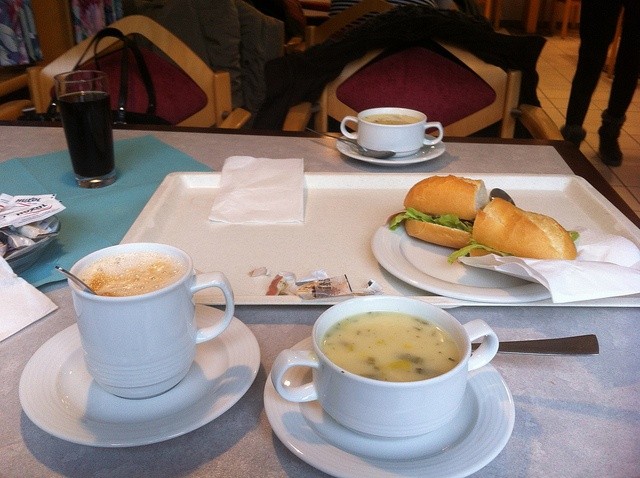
[383,174,580,261]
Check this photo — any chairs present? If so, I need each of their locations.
[281,18,565,141]
[26,15,252,130]
[284,2,393,61]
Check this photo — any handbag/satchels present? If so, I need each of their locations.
[16,27,171,126]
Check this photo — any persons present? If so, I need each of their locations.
[561,0,639,167]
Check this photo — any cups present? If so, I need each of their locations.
[340,108,444,158]
[54,71,118,189]
[68,242,236,399]
[270,296,500,441]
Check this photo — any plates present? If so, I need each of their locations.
[4,215,63,273]
[372,218,551,303]
[336,132,446,167]
[264,335,516,478]
[18,303,260,448]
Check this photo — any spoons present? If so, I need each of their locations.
[488,187,516,207]
[53,265,95,296]
[305,127,396,160]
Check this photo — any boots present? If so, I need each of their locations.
[560,125,586,149]
[598,108,626,166]
